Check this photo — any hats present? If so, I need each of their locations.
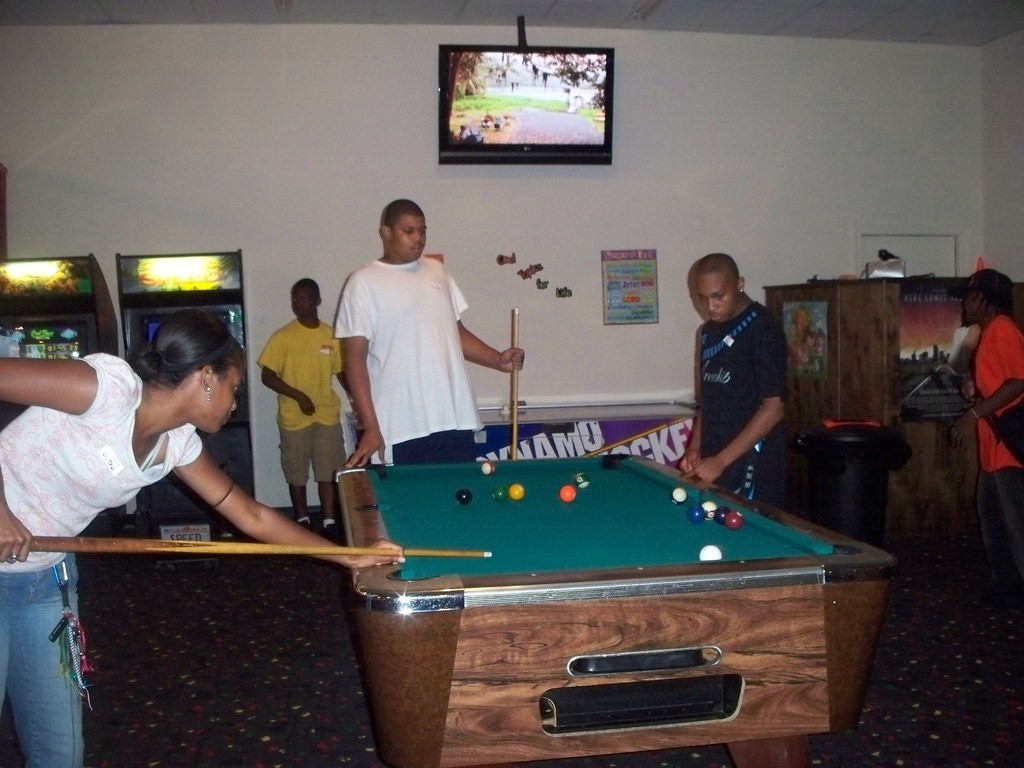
[948,269,1013,301]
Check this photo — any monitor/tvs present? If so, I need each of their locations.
[439,44,615,165]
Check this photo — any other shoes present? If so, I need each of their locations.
[323,523,345,544]
[299,519,311,530]
[974,589,1024,608]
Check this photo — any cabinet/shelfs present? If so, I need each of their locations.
[765,278,1024,541]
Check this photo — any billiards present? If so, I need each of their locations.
[725,512,743,530]
[701,501,717,521]
[560,485,576,503]
[482,462,495,475]
[687,505,705,523]
[508,484,525,500]
[457,489,472,504]
[714,506,731,525]
[492,486,509,501]
[699,545,722,561]
[670,487,688,505]
[575,472,590,489]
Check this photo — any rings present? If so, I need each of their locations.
[7,552,20,561]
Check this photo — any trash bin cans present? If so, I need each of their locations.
[794,420,896,546]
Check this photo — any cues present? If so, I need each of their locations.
[580,411,702,457]
[29,534,493,559]
[512,307,519,460]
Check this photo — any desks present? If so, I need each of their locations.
[335,455,896,768]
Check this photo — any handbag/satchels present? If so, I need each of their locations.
[994,398,1023,467]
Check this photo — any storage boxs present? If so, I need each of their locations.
[867,260,906,279]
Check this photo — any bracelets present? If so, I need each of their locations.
[971,407,980,420]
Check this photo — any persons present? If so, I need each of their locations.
[0,309,406,768]
[679,253,789,511]
[450,110,514,144]
[259,279,355,539]
[960,269,1024,609]
[334,199,526,467]
